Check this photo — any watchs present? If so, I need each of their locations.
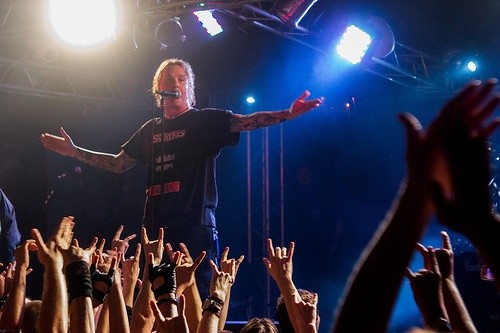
[201,298,221,312]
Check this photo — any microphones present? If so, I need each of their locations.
[156,90,182,99]
[57,167,82,178]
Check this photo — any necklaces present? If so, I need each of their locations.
[164,107,191,120]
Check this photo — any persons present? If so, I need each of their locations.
[0,79,500,333]
[41,59,323,301]
